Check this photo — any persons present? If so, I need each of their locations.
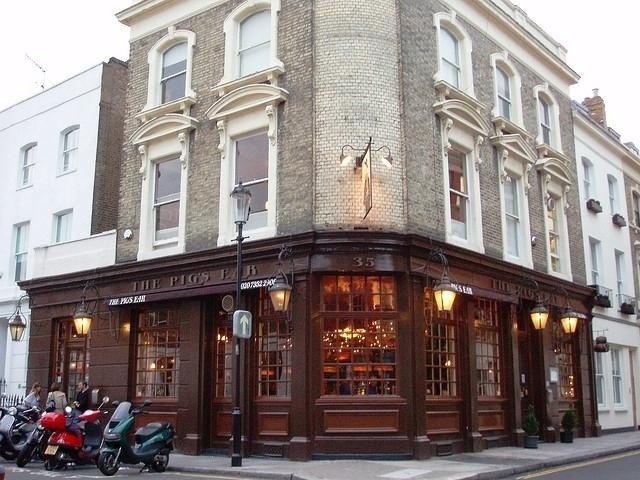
[46,383,67,414]
[23,383,43,410]
[73,381,91,416]
[366,376,382,394]
[339,380,350,394]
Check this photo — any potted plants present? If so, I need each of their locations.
[521,404,540,448]
[560,410,577,443]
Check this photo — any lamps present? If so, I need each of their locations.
[516,278,549,329]
[549,287,579,333]
[340,137,393,169]
[73,281,113,335]
[268,243,299,323]
[424,246,456,327]
[8,295,41,341]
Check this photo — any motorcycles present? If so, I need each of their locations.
[1,383,178,480]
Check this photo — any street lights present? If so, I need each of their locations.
[228,177,256,467]
